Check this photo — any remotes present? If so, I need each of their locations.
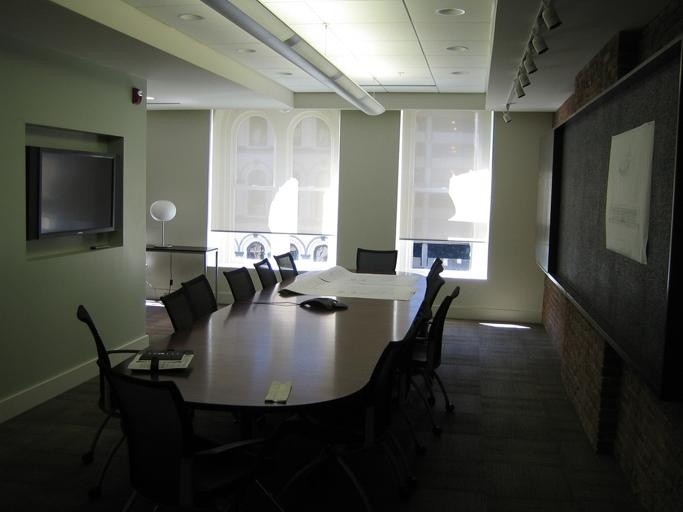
[91,244,111,250]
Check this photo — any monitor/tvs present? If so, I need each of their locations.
[28,145,116,239]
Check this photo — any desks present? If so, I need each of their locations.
[145,242,218,300]
[122,267,432,486]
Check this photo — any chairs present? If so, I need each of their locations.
[114,378,286,511]
[356,249,399,276]
[162,254,299,332]
[76,304,137,465]
[290,342,402,509]
[399,287,460,412]
[424,260,444,321]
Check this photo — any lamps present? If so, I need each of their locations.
[501,2,563,125]
[148,198,175,245]
[199,0,386,118]
